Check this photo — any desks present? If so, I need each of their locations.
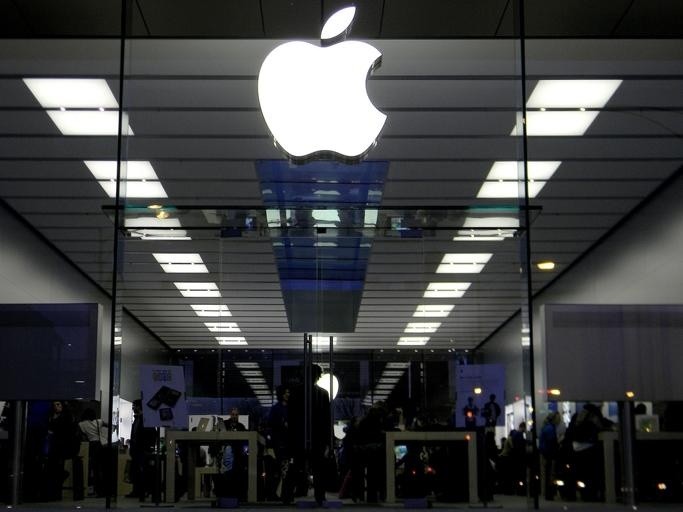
[385,425,482,509]
[163,430,261,506]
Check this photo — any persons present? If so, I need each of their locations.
[462,396,479,429]
[267,364,330,509]
[178,427,207,500]
[75,408,109,499]
[209,407,249,499]
[484,394,502,427]
[338,399,499,509]
[38,402,70,502]
[539,404,647,503]
[499,422,532,496]
[129,398,162,506]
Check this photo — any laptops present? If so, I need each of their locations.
[196,417,210,434]
[216,416,230,432]
[635,416,660,434]
